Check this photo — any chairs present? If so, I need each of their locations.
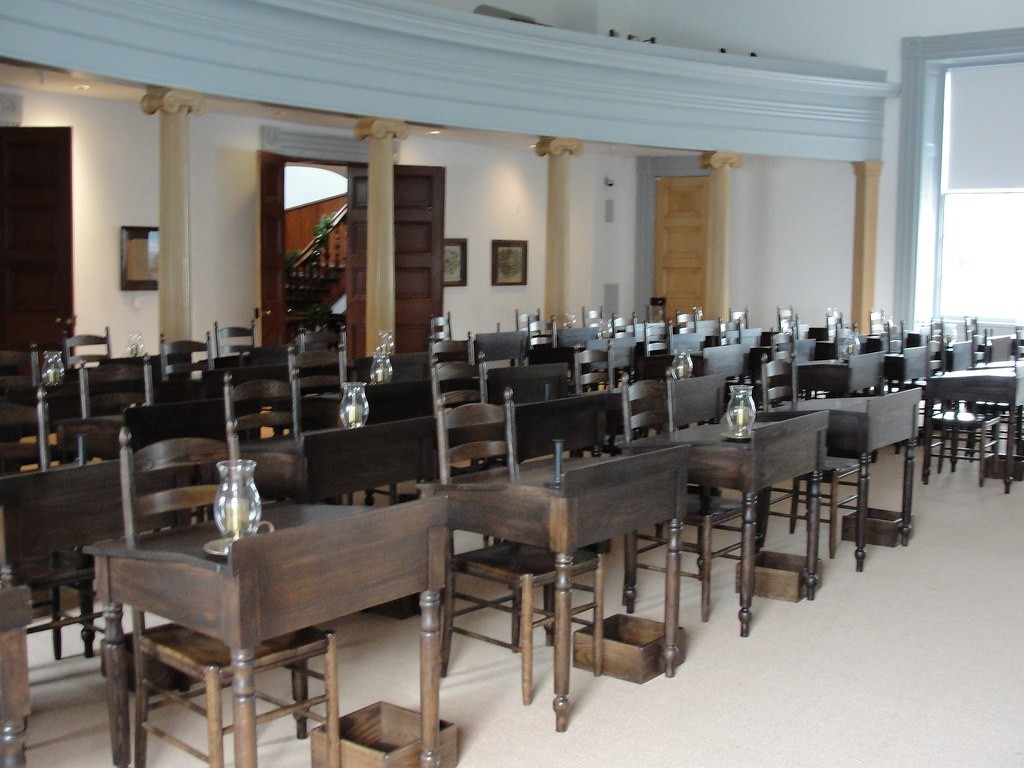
[0,308,1024,768]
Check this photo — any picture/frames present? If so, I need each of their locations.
[442,238,467,287]
[119,226,158,291]
[491,239,530,286]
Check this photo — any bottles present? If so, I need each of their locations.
[376,329,395,355]
[370,352,393,384]
[127,329,144,357]
[671,345,693,380]
[339,381,370,430]
[41,350,65,385]
[597,318,613,340]
[563,314,576,329]
[213,459,262,547]
[726,384,756,436]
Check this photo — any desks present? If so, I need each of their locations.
[432,444,691,732]
[2,461,123,678]
[242,417,439,503]
[556,323,603,350]
[920,364,1024,490]
[797,350,885,397]
[484,360,571,404]
[789,389,924,572]
[987,335,1012,364]
[645,344,749,383]
[945,340,973,370]
[673,372,726,431]
[302,380,433,429]
[57,401,225,457]
[475,328,529,367]
[624,410,829,632]
[447,387,608,457]
[886,345,926,387]
[83,497,448,768]
[586,335,638,382]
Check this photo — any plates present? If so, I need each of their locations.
[720,432,752,441]
[204,539,232,558]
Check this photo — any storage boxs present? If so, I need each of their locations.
[841,508,913,547]
[736,550,821,602]
[312,701,457,768]
[573,613,683,685]
[984,453,1024,481]
